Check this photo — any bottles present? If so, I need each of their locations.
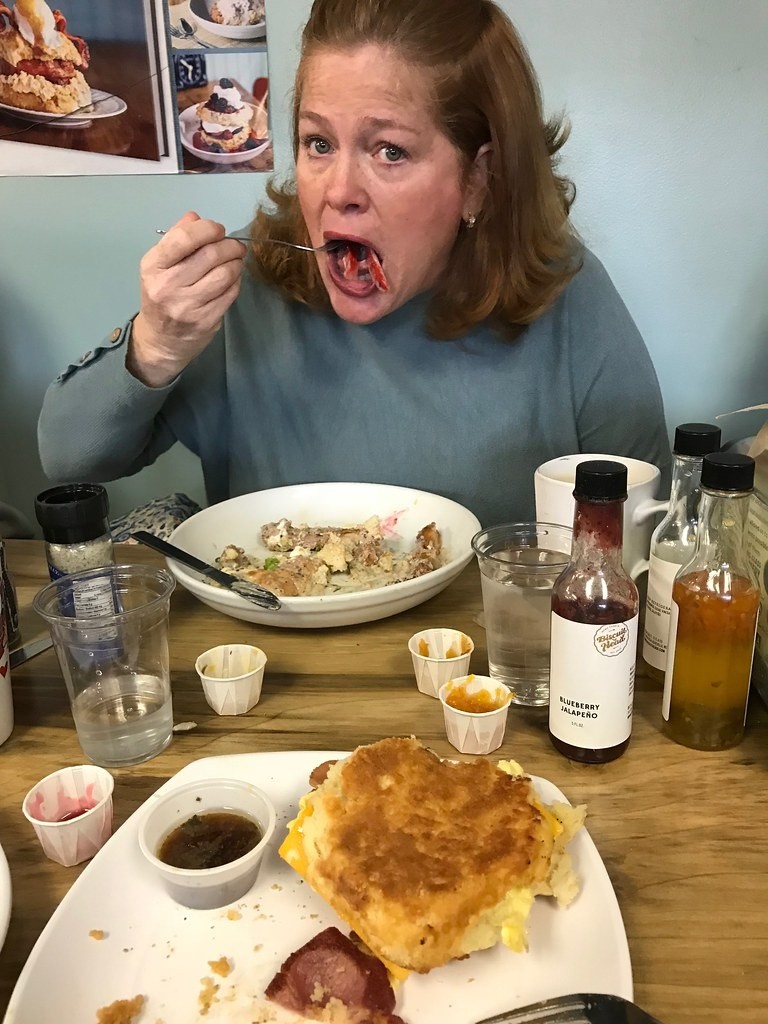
[645,421,723,683]
[661,450,761,753]
[35,484,125,676]
[547,460,639,765]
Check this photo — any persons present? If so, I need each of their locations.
[37,0,672,558]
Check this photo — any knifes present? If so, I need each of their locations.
[130,531,281,612]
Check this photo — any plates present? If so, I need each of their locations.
[1,749,635,1023]
[0,88,128,127]
[0,845,14,953]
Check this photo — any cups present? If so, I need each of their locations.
[534,453,670,583]
[471,522,573,707]
[33,564,177,770]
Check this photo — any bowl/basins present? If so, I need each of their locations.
[21,763,116,868]
[188,0,266,40]
[165,482,484,629]
[178,100,271,164]
[137,777,276,909]
[407,626,474,700]
[194,643,268,716]
[438,675,513,756]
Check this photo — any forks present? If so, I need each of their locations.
[157,229,346,253]
[476,993,662,1024]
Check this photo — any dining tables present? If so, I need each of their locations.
[0,539,768,1024]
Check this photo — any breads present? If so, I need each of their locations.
[277,736,589,974]
